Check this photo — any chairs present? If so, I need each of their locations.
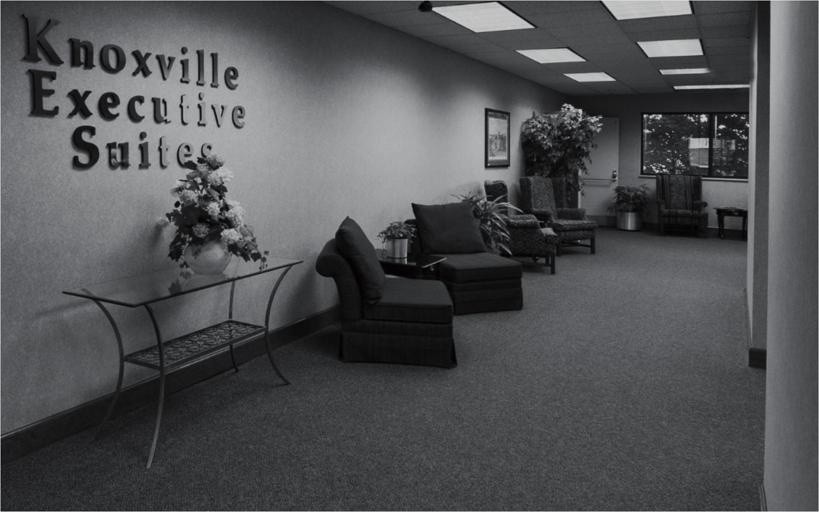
[484,176,598,275]
[655,172,709,239]
[315,200,524,369]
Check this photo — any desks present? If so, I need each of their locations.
[60,254,303,470]
[716,208,747,240]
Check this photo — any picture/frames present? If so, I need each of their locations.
[484,107,511,168]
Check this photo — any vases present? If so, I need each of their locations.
[184,239,232,275]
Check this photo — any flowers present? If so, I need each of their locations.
[165,152,270,271]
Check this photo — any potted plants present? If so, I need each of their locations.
[607,184,650,231]
